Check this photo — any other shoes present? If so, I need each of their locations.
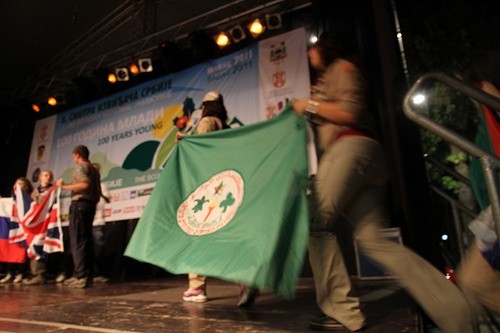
[0,273,12,282]
[23,275,46,285]
[55,274,66,282]
[310,317,346,331]
[13,274,23,282]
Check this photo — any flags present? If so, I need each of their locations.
[123,97,319,301]
[0,179,64,263]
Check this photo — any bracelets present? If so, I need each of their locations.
[305,97,321,115]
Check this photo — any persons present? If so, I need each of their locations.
[290,25,492,333]
[55,162,111,283]
[175,90,258,309]
[0,177,34,283]
[56,144,101,288]
[23,170,54,286]
[456,80,500,319]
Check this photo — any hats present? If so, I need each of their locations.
[199,91,222,109]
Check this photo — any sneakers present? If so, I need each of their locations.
[182,288,207,302]
[93,276,109,283]
[64,277,88,288]
[237,286,255,308]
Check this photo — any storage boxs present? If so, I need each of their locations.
[353,227,405,281]
